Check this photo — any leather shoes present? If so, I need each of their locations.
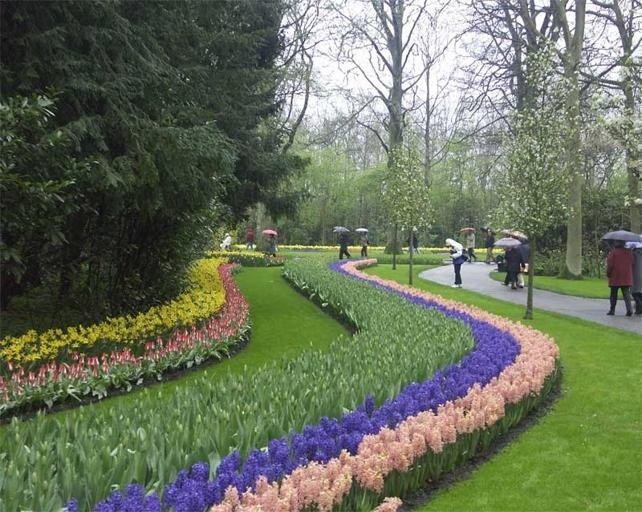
[607,313,615,315]
[626,310,633,316]
[636,310,642,314]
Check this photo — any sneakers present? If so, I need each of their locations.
[501,280,525,290]
[468,257,500,265]
[451,284,459,288]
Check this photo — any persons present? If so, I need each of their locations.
[361,232,368,257]
[406,232,420,254]
[606,241,635,314]
[445,230,529,289]
[337,231,350,259]
[220,227,277,257]
[630,248,642,314]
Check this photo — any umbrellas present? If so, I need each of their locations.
[356,228,368,232]
[480,227,496,235]
[402,224,417,231]
[601,230,642,243]
[624,241,642,248]
[460,228,476,233]
[333,226,350,233]
